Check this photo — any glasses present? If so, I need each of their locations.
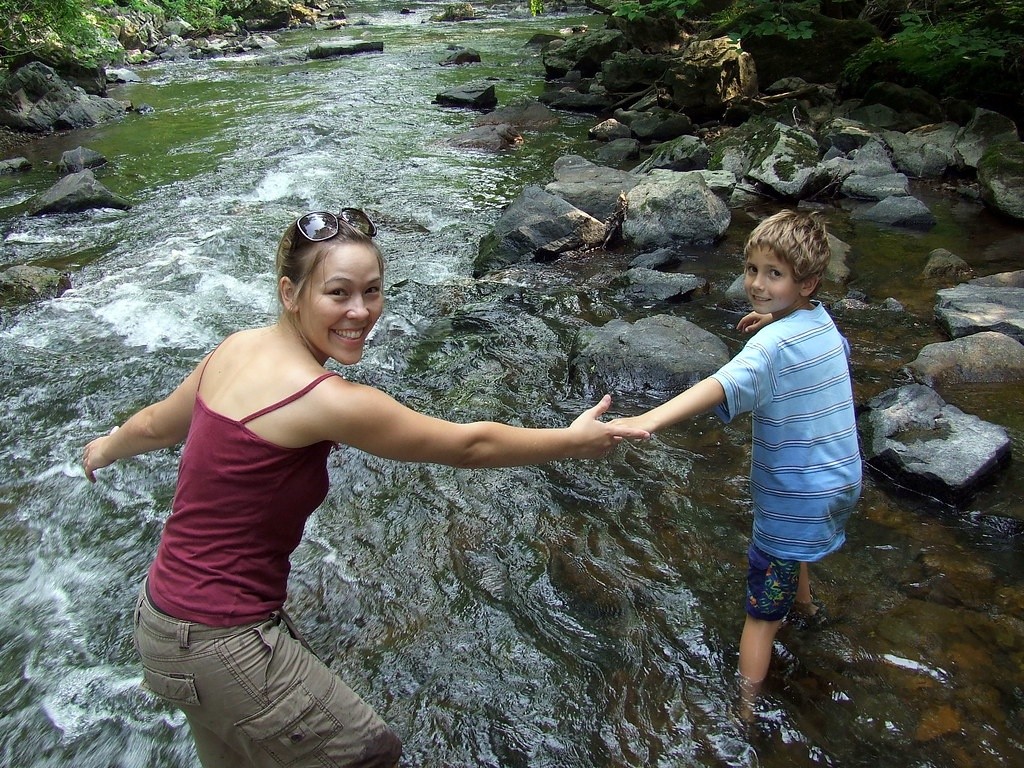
[287,208,377,258]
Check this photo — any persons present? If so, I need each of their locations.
[610,211,862,684]
[80,209,649,768]
